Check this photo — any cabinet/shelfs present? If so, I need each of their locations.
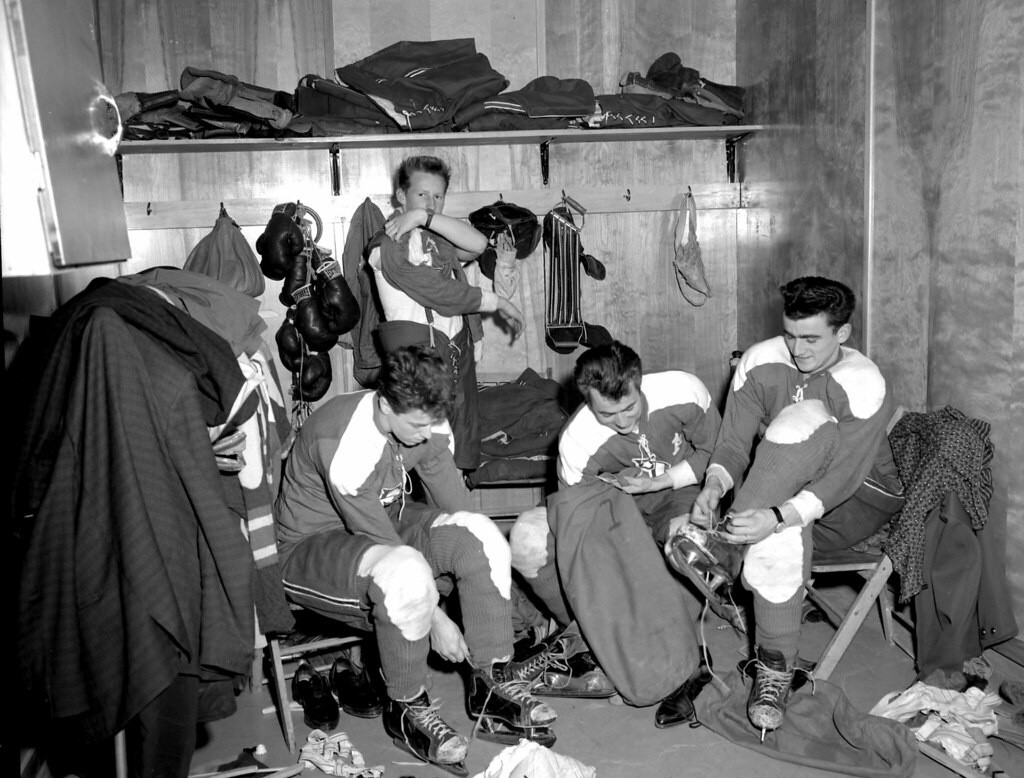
[469,478,557,522]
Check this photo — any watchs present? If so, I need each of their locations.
[769,506,786,533]
[425,208,435,228]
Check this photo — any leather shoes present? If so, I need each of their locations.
[327,653,383,718]
[655,664,714,728]
[292,663,340,732]
[742,659,816,691]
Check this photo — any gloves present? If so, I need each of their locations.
[252,215,362,400]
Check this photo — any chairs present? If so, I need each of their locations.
[266,601,373,758]
[799,411,967,680]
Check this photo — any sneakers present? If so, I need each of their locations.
[385,688,470,778]
[664,512,746,692]
[470,658,558,745]
[747,643,815,743]
[511,617,617,698]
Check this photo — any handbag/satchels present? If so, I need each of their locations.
[545,480,698,706]
[183,206,267,297]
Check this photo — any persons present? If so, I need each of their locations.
[674,277,906,743]
[508,340,744,698]
[273,344,557,777]
[364,157,526,508]
